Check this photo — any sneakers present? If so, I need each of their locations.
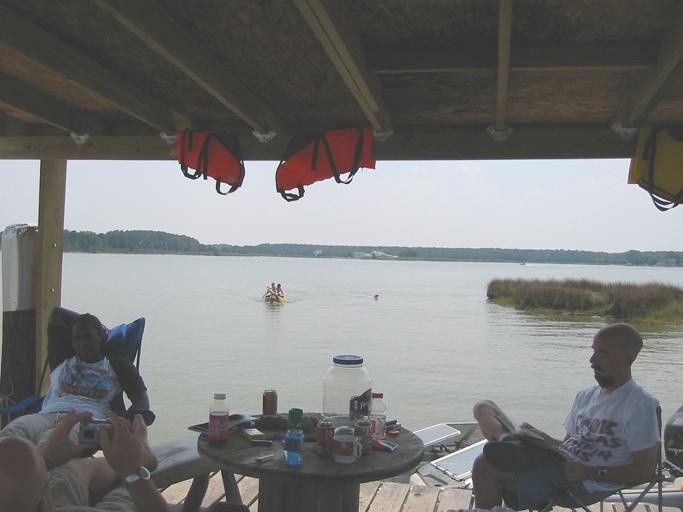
[483,435,551,472]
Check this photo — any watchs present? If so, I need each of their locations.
[597,465,608,483]
[122,465,153,484]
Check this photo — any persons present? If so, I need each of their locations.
[0,408,173,512]
[267,282,275,295]
[274,284,282,293]
[446,324,662,512]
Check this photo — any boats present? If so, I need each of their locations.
[262,295,285,306]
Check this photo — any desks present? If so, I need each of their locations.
[198,412,424,512]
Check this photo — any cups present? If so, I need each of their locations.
[332,434,362,464]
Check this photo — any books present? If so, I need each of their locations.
[494,411,572,459]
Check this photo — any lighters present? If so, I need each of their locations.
[249,440,273,445]
[255,454,276,465]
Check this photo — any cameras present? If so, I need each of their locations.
[78,417,116,450]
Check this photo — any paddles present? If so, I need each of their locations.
[269,288,284,304]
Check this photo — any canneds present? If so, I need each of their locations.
[263,389,277,416]
[355,422,371,455]
[317,422,335,457]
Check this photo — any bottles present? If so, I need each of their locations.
[208,392,230,442]
[321,354,372,423]
[368,392,387,439]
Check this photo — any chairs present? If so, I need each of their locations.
[540,407,665,512]
